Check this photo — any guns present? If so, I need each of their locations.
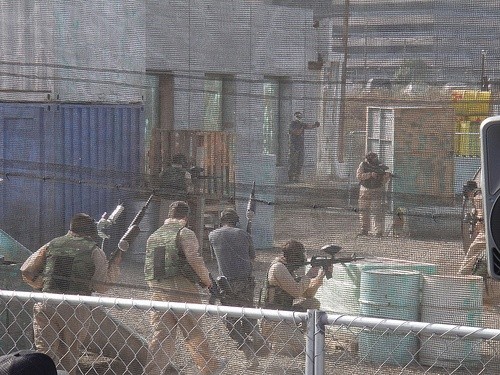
[109,194,154,269]
[287,244,365,280]
[246,182,256,234]
[365,165,399,181]
[182,262,234,306]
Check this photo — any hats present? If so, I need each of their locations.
[0,349,58,375]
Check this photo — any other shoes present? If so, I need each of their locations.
[358,230,368,236]
[243,344,258,371]
[208,357,228,375]
[289,177,300,183]
[376,232,382,237]
[254,339,269,355]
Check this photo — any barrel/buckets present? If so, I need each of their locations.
[419,274,484,369]
[358,269,422,365]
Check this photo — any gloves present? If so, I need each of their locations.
[315,121,320,127]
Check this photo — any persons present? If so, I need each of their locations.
[143,200,228,375]
[19,212,121,375]
[259,240,334,356]
[457,180,486,276]
[0,349,58,375]
[287,111,320,183]
[357,153,394,238]
[160,153,193,230]
[208,209,270,371]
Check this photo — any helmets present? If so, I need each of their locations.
[281,239,305,256]
[169,200,190,215]
[462,180,477,199]
[220,208,240,227]
[366,152,379,167]
